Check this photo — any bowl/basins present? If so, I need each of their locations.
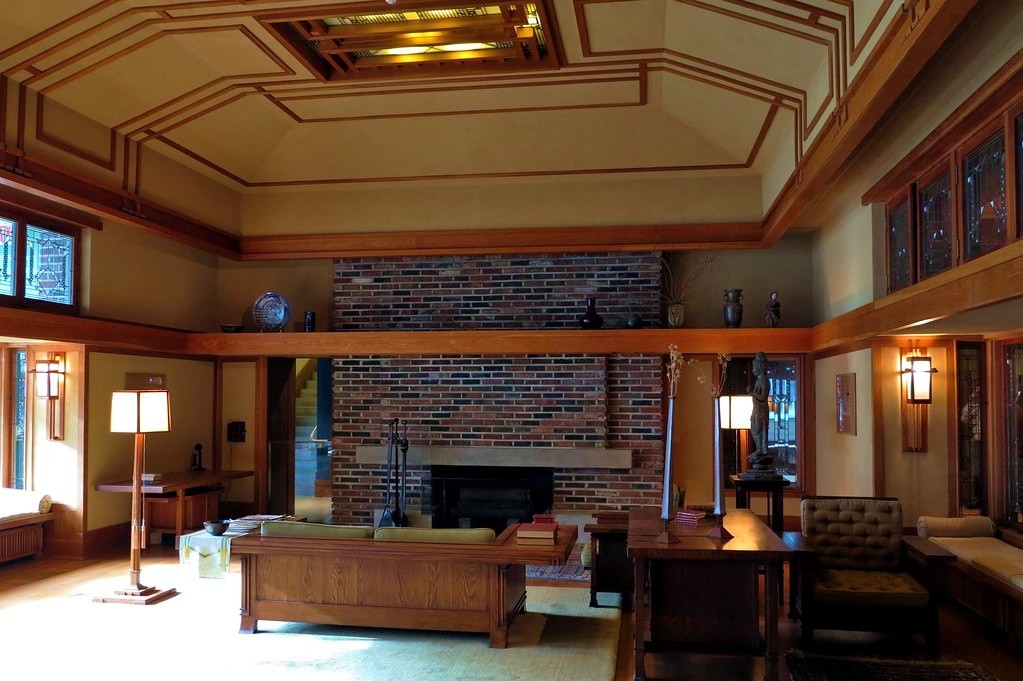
[220,323,246,332]
[202,520,230,536]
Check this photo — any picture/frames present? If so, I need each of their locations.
[834,373,857,435]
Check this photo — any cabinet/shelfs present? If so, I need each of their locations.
[141,486,227,545]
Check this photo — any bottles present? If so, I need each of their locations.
[723,288,744,328]
[578,296,603,330]
[625,314,641,329]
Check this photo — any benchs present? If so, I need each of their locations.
[917,515,1023,652]
[0,482,56,568]
[174,515,578,649]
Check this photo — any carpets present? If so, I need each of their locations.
[785,653,1000,681]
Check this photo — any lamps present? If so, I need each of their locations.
[28,351,65,442]
[902,352,937,405]
[717,393,755,473]
[91,388,178,605]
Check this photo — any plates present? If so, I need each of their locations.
[251,291,291,332]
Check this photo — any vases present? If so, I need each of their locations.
[580,287,746,330]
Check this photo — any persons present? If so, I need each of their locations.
[745,351,771,455]
[765,292,781,328]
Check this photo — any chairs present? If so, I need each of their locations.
[779,495,959,657]
[583,482,685,610]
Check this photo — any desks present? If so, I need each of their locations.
[729,474,791,531]
[95,468,254,551]
[626,505,793,681]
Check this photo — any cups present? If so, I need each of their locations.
[304,311,316,332]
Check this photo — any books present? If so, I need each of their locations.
[516,513,559,546]
[132,473,162,485]
[677,501,715,526]
